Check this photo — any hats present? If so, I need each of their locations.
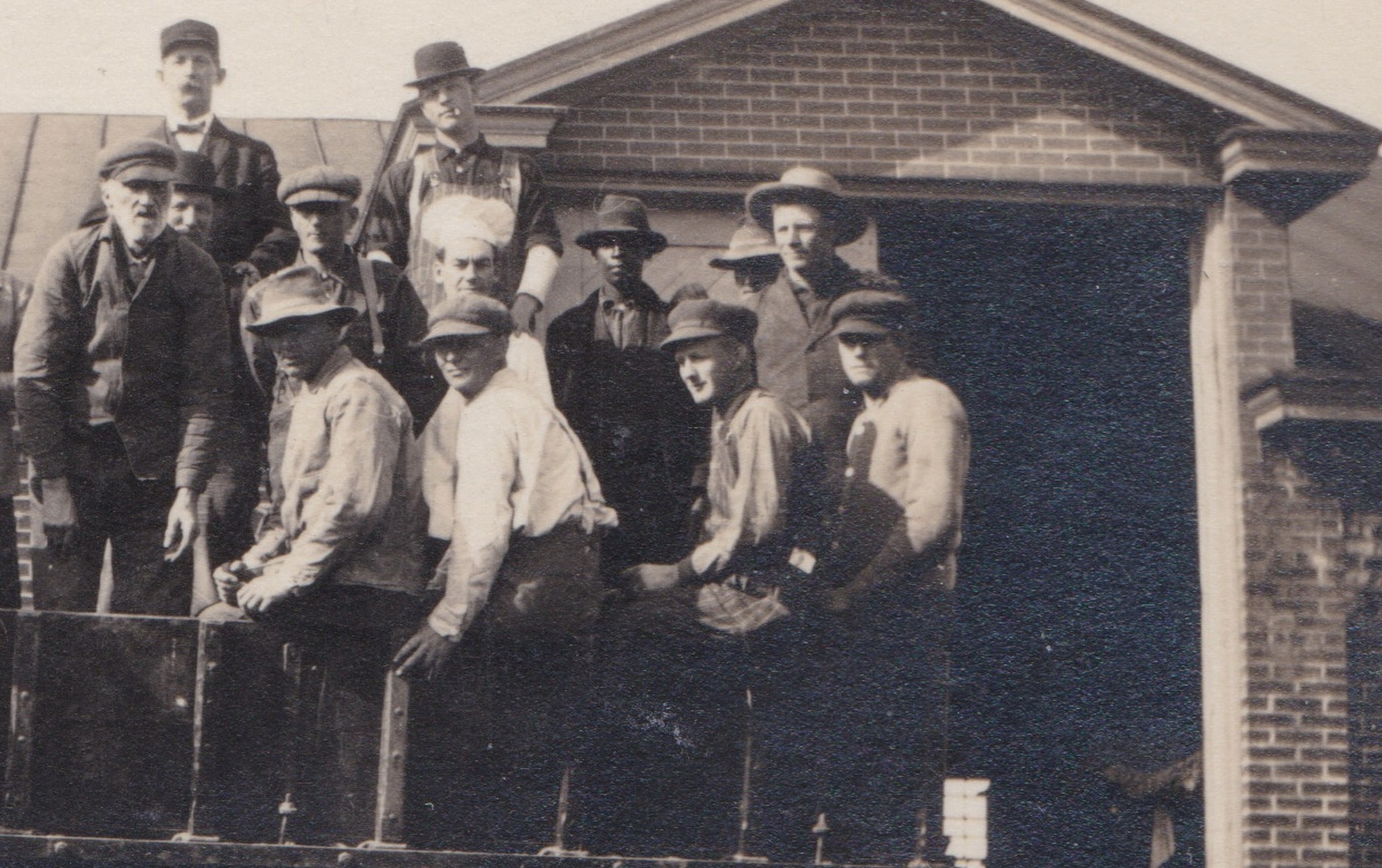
[421,296,511,343]
[94,135,177,184]
[247,264,356,337]
[659,298,760,350]
[421,194,516,250]
[160,18,220,60]
[742,166,871,249]
[821,290,918,344]
[172,151,229,197]
[403,40,485,95]
[708,227,780,269]
[275,167,364,203]
[575,196,668,255]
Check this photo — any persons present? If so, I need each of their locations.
[407,195,557,564]
[168,149,263,563]
[813,292,970,616]
[547,195,707,589]
[277,165,429,423]
[195,264,430,623]
[393,292,604,679]
[737,165,902,555]
[708,218,780,306]
[596,300,819,648]
[15,137,232,615]
[75,18,300,283]
[362,40,564,341]
